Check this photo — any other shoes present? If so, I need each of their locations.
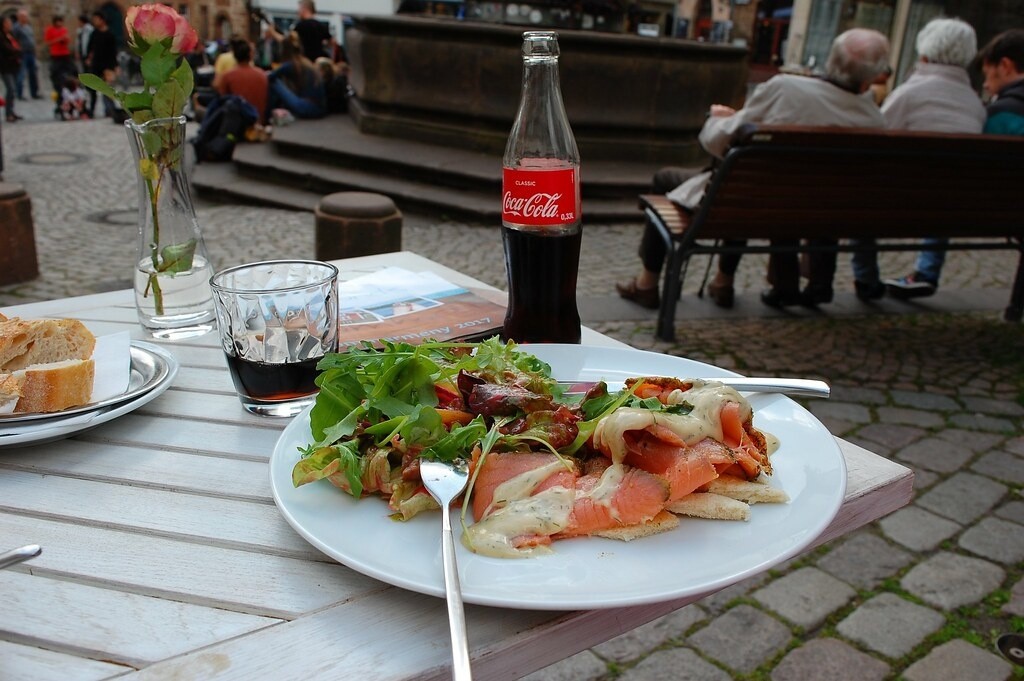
[616,278,659,310]
[707,283,733,308]
[854,275,881,303]
[800,279,833,307]
[881,273,936,300]
[761,287,801,306]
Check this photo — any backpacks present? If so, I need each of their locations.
[190,98,256,164]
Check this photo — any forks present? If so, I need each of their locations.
[419,453,501,681]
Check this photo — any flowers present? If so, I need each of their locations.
[80,1,200,316]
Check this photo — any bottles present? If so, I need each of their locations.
[500,29,583,344]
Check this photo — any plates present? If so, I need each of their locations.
[269,343,848,611]
[0,339,181,447]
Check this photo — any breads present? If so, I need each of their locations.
[0,358,95,417]
[0,310,96,371]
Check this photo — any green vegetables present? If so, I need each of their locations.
[296,337,694,500]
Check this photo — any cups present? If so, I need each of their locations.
[210,258,341,421]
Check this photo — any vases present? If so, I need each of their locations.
[124,114,219,330]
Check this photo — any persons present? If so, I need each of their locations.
[850,29,1024,302]
[760,18,988,308]
[617,28,889,314]
[190,0,348,128]
[43,10,120,121]
[0,10,44,123]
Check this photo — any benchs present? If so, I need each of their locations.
[639,121,1024,341]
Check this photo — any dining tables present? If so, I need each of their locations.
[0,249,916,681]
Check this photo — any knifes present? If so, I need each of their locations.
[555,376,829,401]
